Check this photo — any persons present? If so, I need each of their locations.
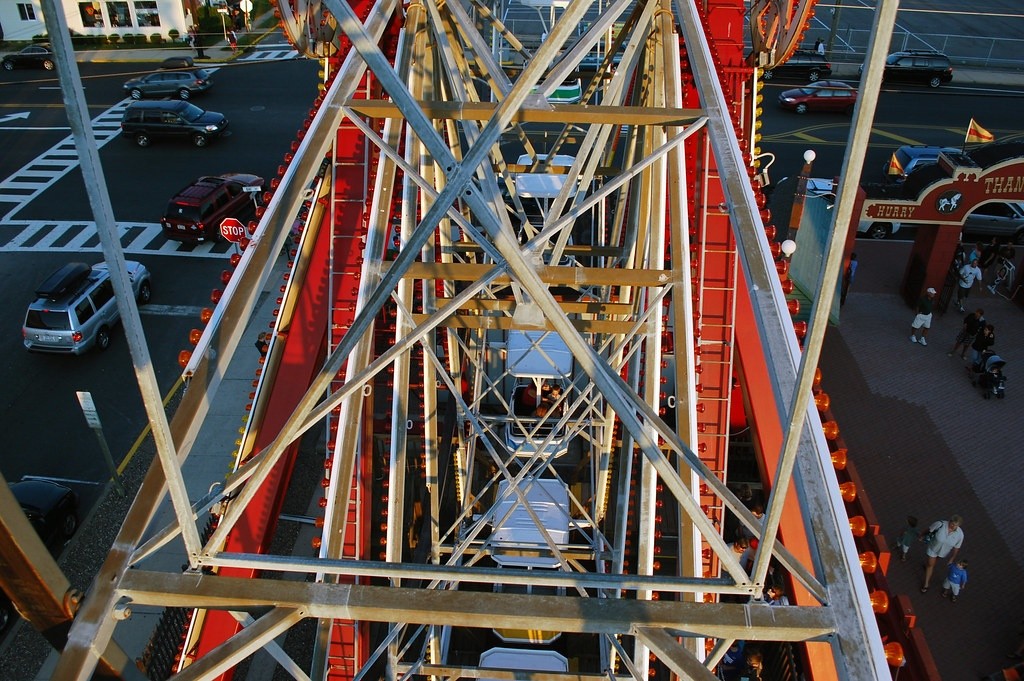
[890,516,922,562]
[911,288,937,345]
[186,24,201,54]
[917,514,964,593]
[941,558,969,602]
[844,252,858,300]
[957,259,982,312]
[719,640,764,681]
[225,30,238,54]
[990,367,1005,395]
[719,482,789,605]
[947,308,995,371]
[814,37,825,55]
[254,331,268,357]
[522,378,563,435]
[959,236,1016,280]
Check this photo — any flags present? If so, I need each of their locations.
[965,117,994,143]
[888,152,905,176]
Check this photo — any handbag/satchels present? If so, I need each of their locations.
[923,521,944,544]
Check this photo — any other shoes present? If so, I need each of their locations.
[961,355,967,360]
[900,553,906,561]
[948,351,954,356]
[920,584,928,593]
[959,306,966,312]
[892,544,898,550]
[952,596,957,602]
[909,334,917,343]
[918,337,927,346]
[942,591,948,598]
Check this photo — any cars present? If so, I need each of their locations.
[778,79,857,113]
[776,177,900,240]
[0,477,83,635]
[964,202,1024,240]
[2,42,55,71]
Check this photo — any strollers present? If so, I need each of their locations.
[971,350,1008,399]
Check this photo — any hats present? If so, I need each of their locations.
[927,288,937,294]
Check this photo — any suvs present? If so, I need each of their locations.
[120,101,229,147]
[881,144,966,183]
[21,258,152,355]
[160,171,265,242]
[123,68,215,100]
[762,52,832,82]
[857,51,953,88]
